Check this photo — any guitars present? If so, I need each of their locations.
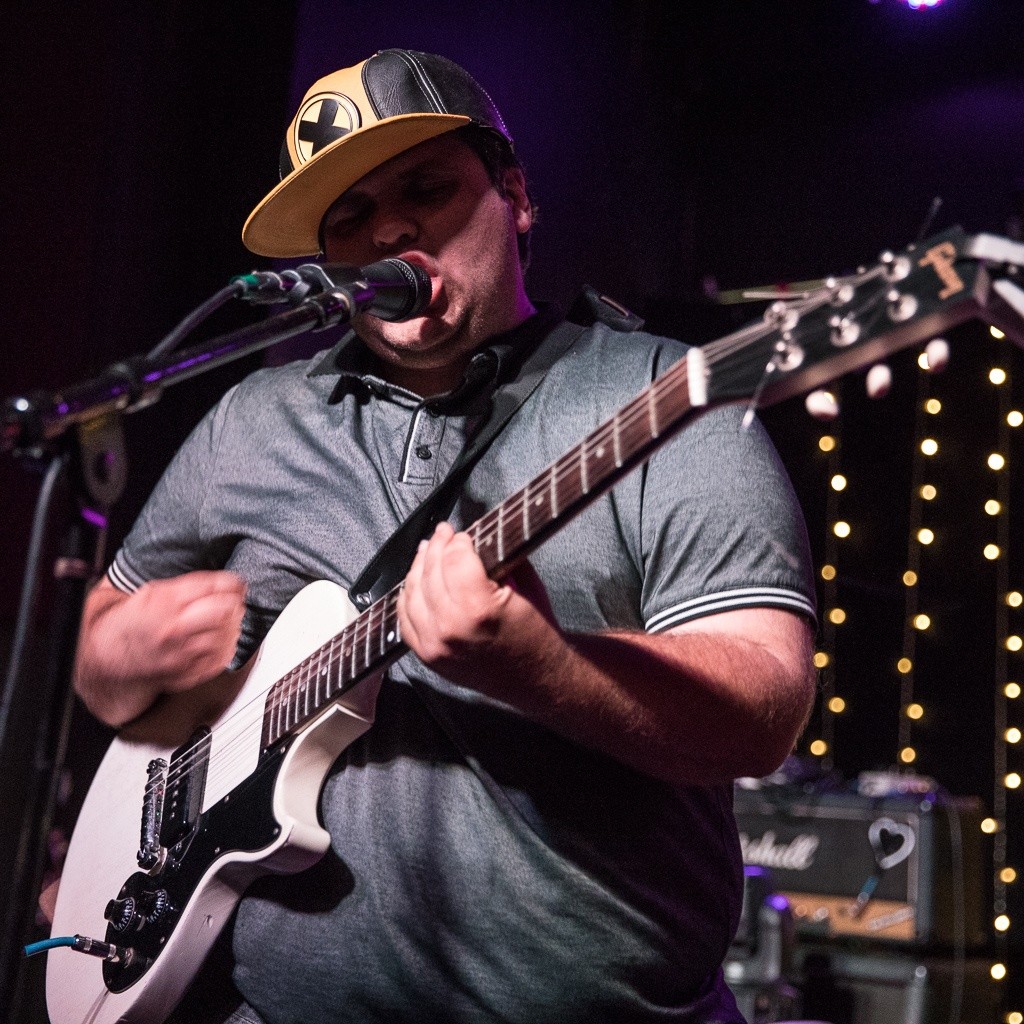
[38,215,997,1024]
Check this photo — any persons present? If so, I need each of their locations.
[72,48,820,1024]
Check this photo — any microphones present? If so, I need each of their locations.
[233,257,432,325]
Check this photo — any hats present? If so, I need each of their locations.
[241,48,513,259]
